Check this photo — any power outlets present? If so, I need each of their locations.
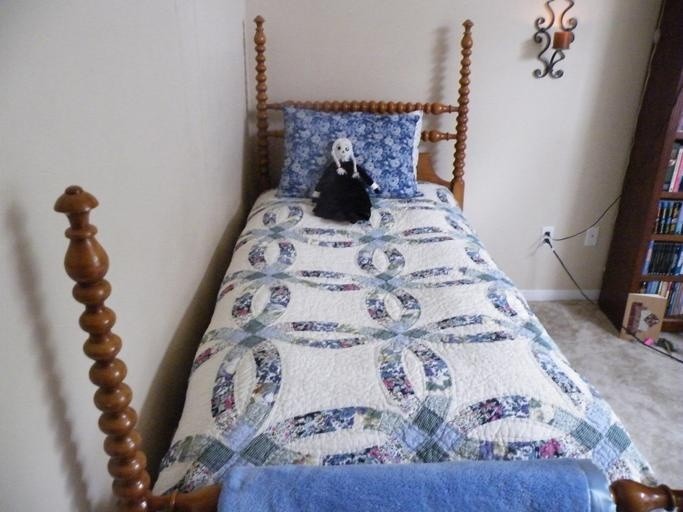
[540,226,555,247]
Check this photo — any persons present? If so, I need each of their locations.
[310,136,383,224]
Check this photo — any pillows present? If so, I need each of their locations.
[276,106,423,199]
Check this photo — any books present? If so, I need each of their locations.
[634,109,683,320]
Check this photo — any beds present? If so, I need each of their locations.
[53,16,683,512]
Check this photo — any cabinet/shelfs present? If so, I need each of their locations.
[598,0,683,335]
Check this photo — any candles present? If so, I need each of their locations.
[554,30,570,49]
